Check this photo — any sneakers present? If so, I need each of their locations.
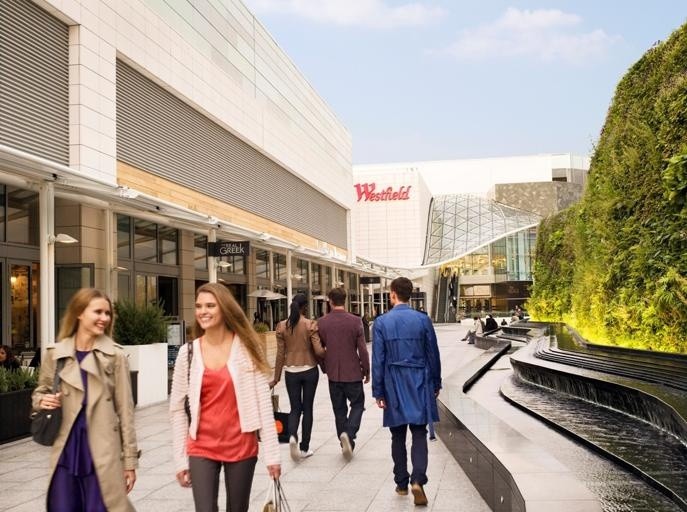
[340,432,353,460]
[396,486,408,495]
[300,449,313,457]
[289,435,300,463]
[411,483,428,505]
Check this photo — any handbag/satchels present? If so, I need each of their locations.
[29,359,66,446]
[274,411,289,442]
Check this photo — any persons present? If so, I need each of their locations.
[0,343,21,372]
[266,290,327,464]
[167,281,281,511]
[460,305,522,345]
[361,310,371,343]
[441,269,456,322]
[314,286,371,461]
[368,276,442,507]
[30,287,138,512]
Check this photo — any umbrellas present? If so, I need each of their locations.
[263,294,287,302]
[312,294,329,301]
[246,289,270,298]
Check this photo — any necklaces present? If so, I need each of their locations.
[73,343,93,350]
